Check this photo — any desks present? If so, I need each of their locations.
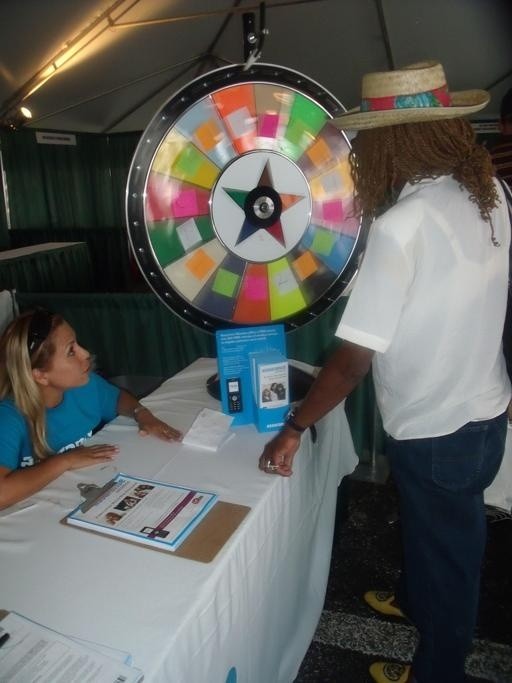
[1,357,360,683]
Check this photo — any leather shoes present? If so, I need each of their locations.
[365,587,403,616]
[369,662,409,681]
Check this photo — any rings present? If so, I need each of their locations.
[268,464,280,471]
[264,458,271,466]
[162,429,168,434]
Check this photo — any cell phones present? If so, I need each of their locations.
[227,378,243,412]
[139,526,170,539]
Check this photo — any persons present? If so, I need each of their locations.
[103,484,153,525]
[257,59,512,681]
[480,88,512,190]
[0,308,184,515]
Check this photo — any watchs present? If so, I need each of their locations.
[282,404,305,432]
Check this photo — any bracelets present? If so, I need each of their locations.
[132,404,147,423]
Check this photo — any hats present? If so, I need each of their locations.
[328,62,489,130]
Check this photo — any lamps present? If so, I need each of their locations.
[10,106,33,132]
